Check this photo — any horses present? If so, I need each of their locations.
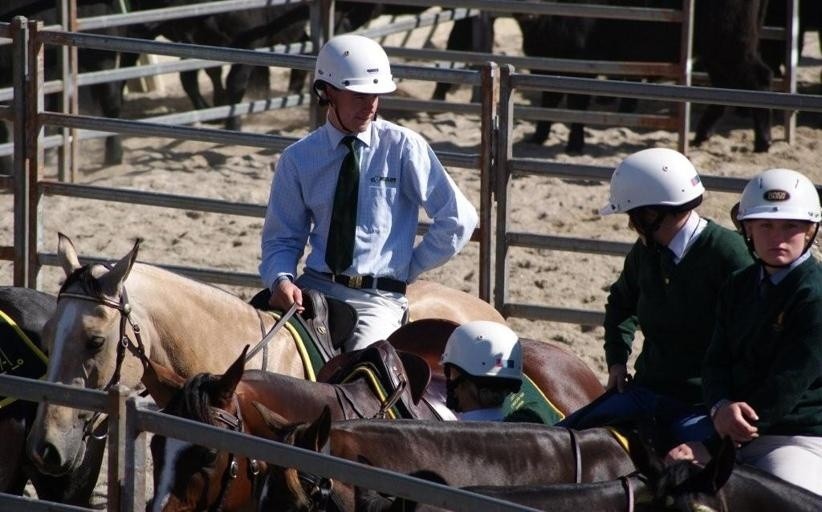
[141,334,606,511]
[24,231,508,479]
[249,399,636,511]
[0,3,490,187]
[514,1,822,156]
[354,446,654,511]
[0,285,108,507]
[644,434,822,511]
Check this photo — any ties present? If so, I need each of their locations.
[325,137,359,274]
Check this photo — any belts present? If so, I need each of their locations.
[320,271,406,294]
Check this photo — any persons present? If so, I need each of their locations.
[660,167,821,499]
[254,33,482,358]
[547,147,760,428]
[437,320,525,423]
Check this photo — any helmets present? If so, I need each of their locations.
[312,35,397,95]
[601,147,704,215]
[438,321,522,384]
[736,169,820,222]
